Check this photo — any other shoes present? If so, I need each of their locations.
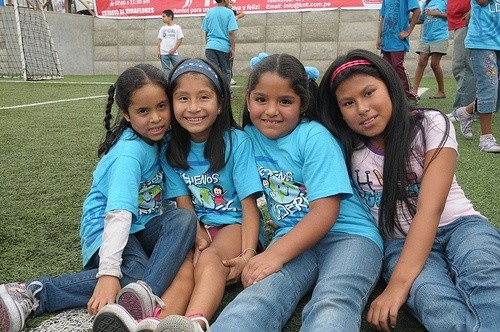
[156,314,210,332]
[134,317,160,332]
[231,79,236,85]
[429,91,447,99]
[446,113,460,122]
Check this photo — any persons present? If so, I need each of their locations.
[157,10,184,84]
[0,64,197,332]
[135,58,263,332]
[92,52,384,332]
[446,0,500,152]
[202,0,245,87]
[376,0,421,100]
[315,49,500,332]
[409,0,448,100]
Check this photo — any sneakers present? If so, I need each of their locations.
[0,280,44,332]
[456,107,477,139]
[92,302,161,332]
[116,279,165,320]
[479,134,500,152]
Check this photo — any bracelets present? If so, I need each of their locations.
[242,248,257,255]
[242,253,247,264]
